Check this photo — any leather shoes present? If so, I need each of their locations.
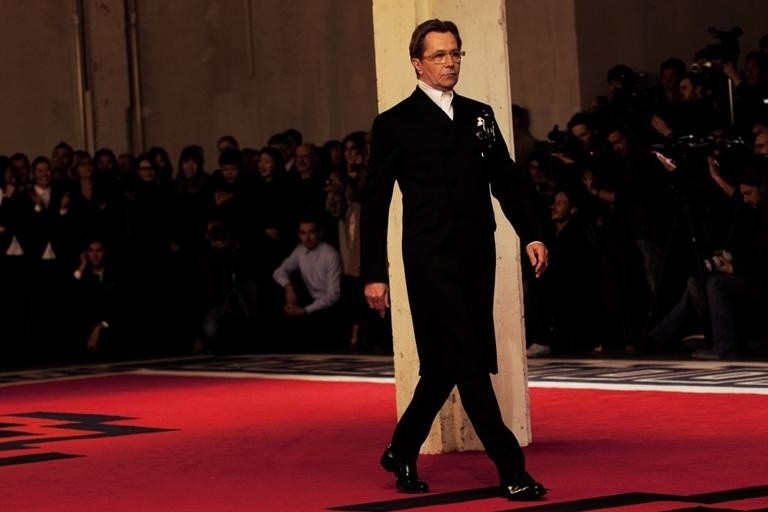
[499,470,547,501]
[379,442,429,493]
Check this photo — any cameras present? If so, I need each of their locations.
[704,250,732,271]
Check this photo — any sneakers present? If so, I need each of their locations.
[691,346,740,361]
[526,340,557,359]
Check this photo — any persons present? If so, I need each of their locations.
[0,126,392,369]
[358,16,555,504]
[506,23,767,365]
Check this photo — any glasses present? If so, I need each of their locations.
[421,49,465,65]
